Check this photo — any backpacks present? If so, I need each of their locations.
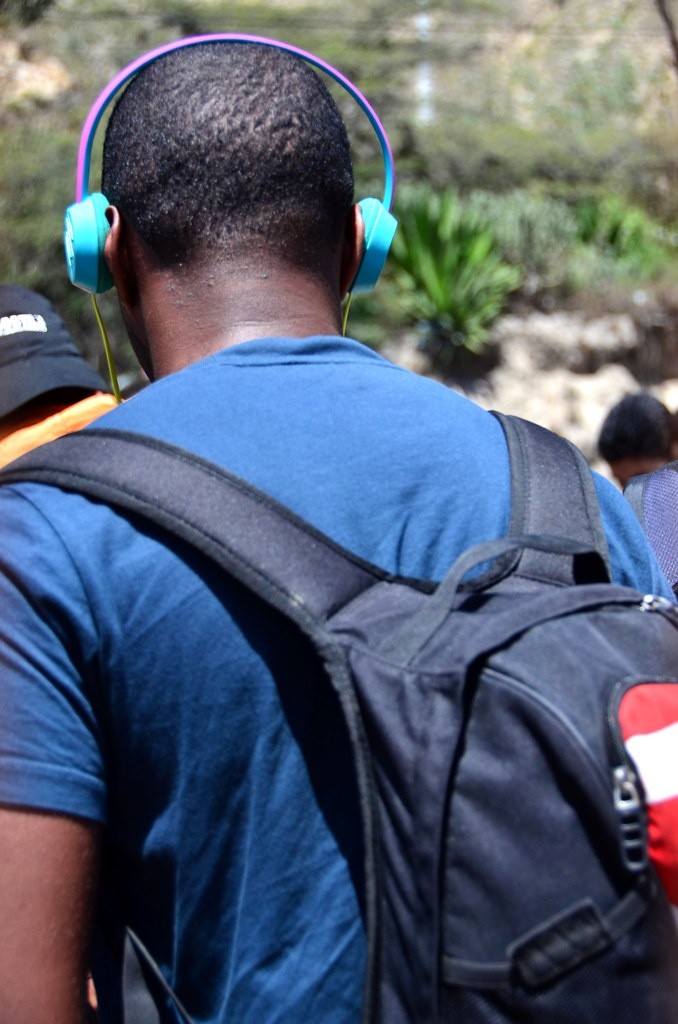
[1,404,677,1024]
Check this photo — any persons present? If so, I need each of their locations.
[0,33,677,1024]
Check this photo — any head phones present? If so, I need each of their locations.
[64,33,397,296]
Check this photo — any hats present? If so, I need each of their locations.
[0,288,113,421]
